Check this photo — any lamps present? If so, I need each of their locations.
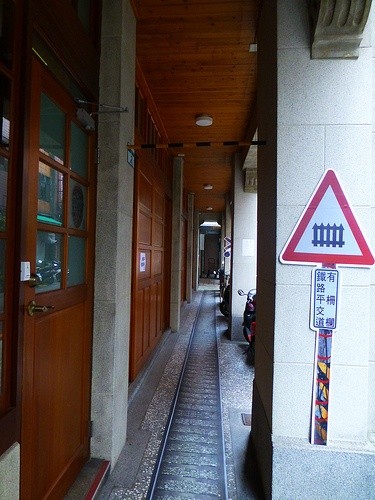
[204,184,213,189]
[196,115,214,127]
[206,206,213,210]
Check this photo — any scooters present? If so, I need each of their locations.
[36,257,70,284]
[237,287,255,365]
